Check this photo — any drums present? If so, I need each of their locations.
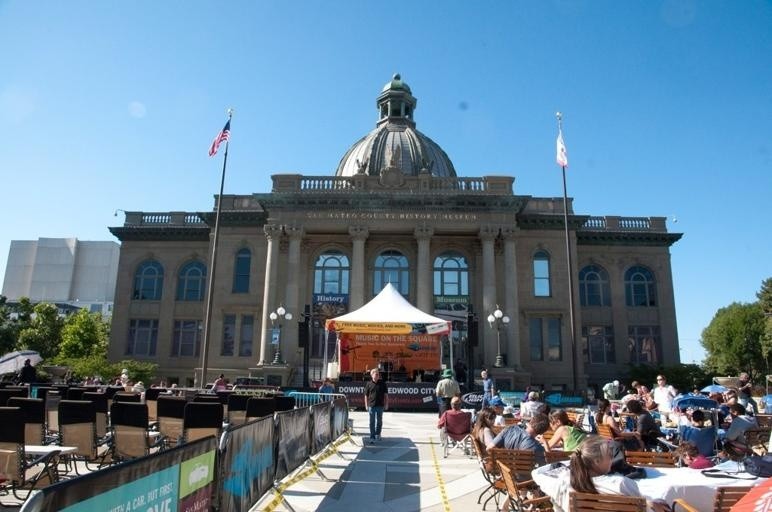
[383,360,393,371]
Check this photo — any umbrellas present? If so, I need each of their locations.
[1,350,44,376]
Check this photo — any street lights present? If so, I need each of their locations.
[486,305,511,367]
[269,302,294,365]
[744,335,752,377]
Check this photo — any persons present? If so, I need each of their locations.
[319,378,335,401]
[20,358,180,396]
[363,368,388,444]
[211,373,226,392]
[435,369,771,511]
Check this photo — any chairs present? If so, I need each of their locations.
[1,374,296,507]
[440,390,771,512]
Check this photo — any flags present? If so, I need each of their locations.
[209,115,230,157]
[556,128,567,168]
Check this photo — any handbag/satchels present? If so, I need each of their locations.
[744,453,772,477]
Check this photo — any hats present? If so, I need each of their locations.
[441,369,452,378]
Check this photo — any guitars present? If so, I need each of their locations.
[342,343,362,354]
